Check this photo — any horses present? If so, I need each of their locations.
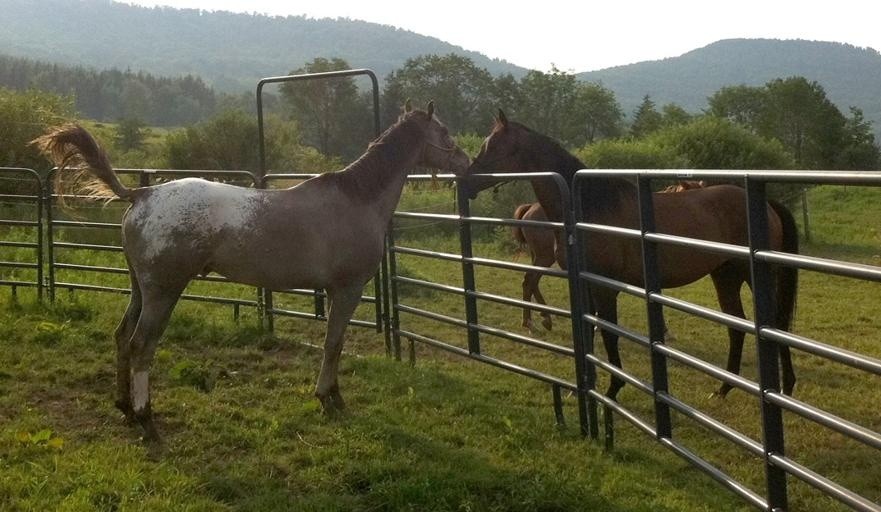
[28,96,470,462]
[513,180,708,330]
[459,108,799,402]
